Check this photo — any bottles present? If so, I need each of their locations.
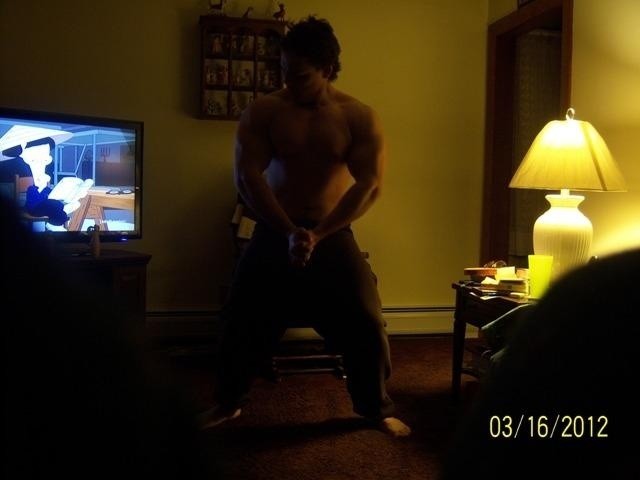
[91,226,100,256]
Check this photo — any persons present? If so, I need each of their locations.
[196,15,412,438]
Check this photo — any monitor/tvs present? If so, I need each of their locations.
[0,105,144,251]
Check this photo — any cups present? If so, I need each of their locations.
[528,255,553,296]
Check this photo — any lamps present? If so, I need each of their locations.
[507,108,627,281]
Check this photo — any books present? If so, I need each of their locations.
[48,177,93,203]
[464,267,527,298]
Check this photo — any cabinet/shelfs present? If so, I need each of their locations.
[1,250,154,379]
[193,13,288,122]
